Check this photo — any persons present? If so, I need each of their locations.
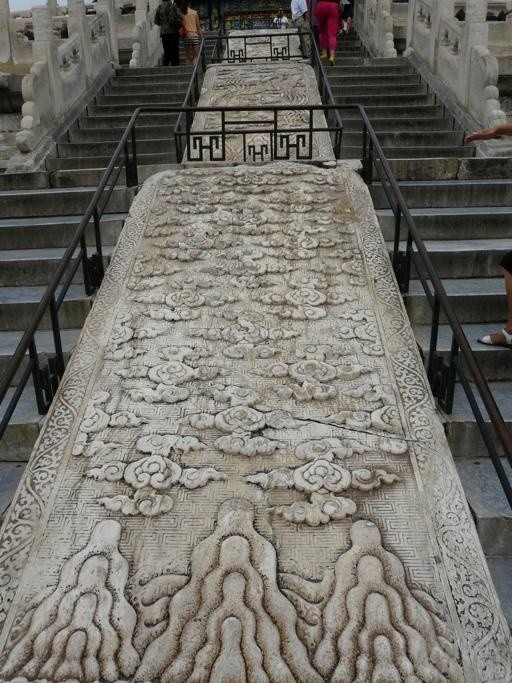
[461,120,511,348]
[314,0,341,67]
[154,0,182,66]
[177,0,204,65]
[289,0,312,58]
[340,0,355,35]
[272,7,290,28]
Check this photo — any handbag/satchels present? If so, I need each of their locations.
[178,25,187,38]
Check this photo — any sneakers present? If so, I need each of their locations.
[329,56,335,66]
[319,53,327,63]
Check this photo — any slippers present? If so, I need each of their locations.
[479,327,512,347]
